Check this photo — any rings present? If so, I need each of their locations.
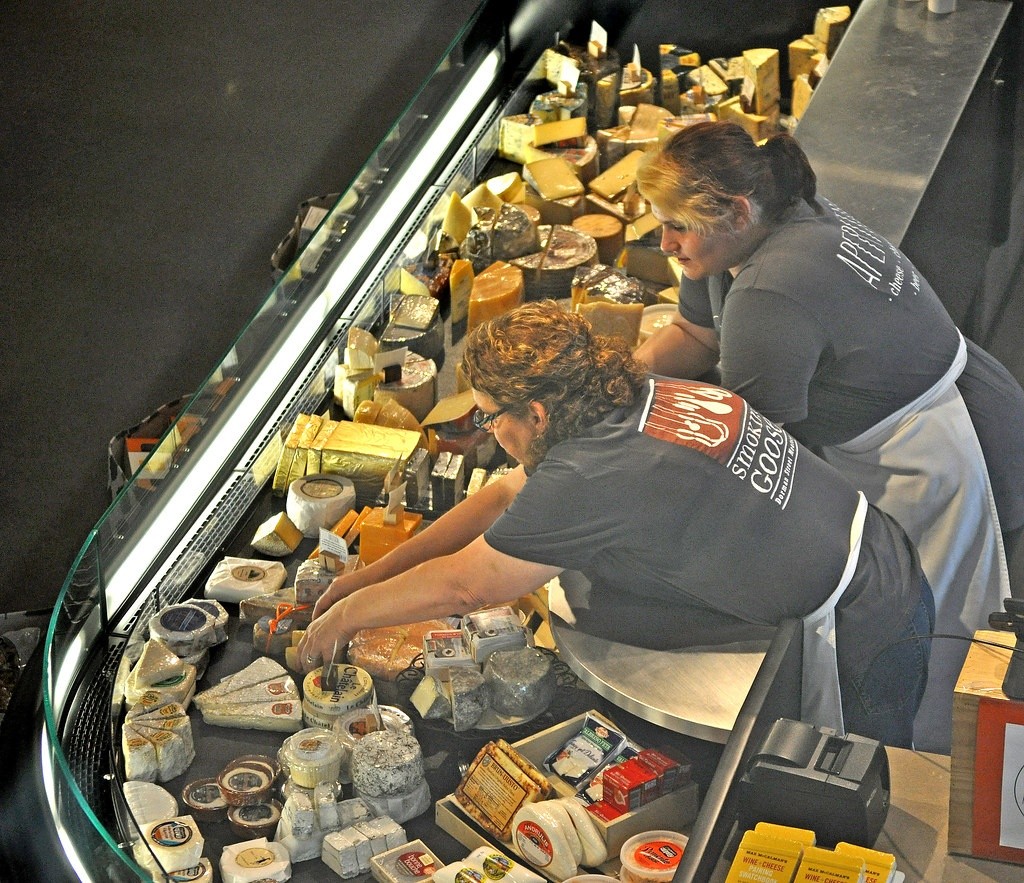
[307,656,318,663]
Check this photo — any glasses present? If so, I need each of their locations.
[473,404,510,434]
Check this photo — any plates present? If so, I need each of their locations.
[443,706,546,729]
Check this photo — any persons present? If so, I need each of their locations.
[296,301,937,746]
[627,120,1024,754]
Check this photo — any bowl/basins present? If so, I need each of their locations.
[182,756,284,840]
[561,831,688,883]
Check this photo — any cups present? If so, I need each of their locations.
[927,0,956,14]
